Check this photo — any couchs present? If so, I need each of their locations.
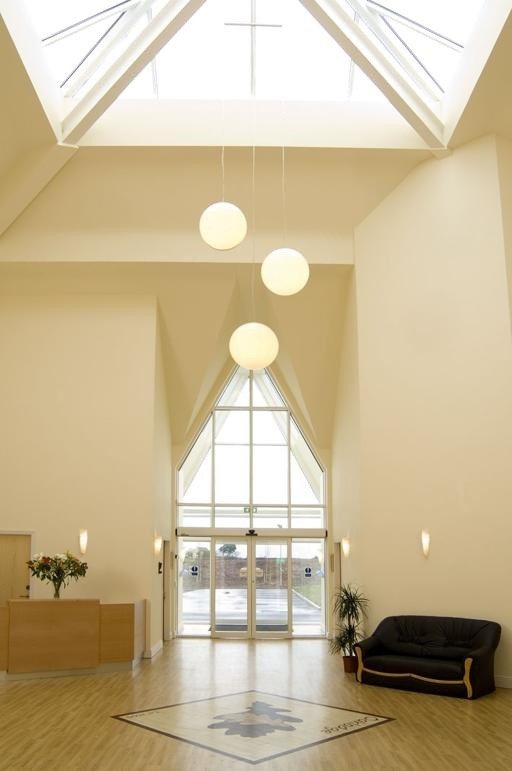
[351,615,502,700]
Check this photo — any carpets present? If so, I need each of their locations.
[105,688,396,769]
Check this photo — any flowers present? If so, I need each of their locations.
[23,550,88,583]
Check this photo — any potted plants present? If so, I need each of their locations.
[327,583,370,672]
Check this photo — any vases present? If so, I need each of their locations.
[54,579,60,598]
